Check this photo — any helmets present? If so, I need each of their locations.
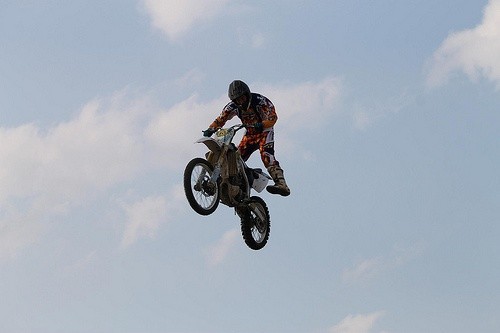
[228,80,251,108]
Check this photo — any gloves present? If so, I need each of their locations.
[253,122,263,133]
[203,129,211,137]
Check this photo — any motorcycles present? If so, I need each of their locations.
[183,123,271,250]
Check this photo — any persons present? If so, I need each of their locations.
[202,80,292,197]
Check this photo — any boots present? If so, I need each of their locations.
[267,164,290,196]
